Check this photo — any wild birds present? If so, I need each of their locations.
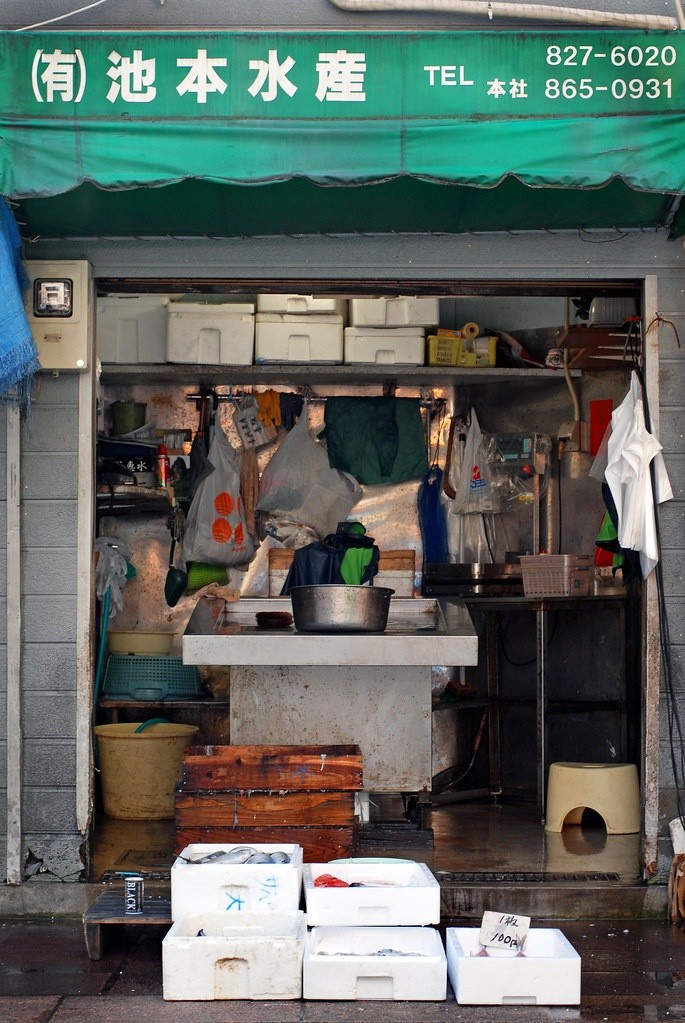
[196,929,206,936]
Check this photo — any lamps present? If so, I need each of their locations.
[587,296,636,328]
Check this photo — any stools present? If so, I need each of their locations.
[544,761,642,835]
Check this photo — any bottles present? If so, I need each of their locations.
[588,568,603,595]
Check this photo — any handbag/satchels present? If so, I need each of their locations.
[453,407,513,516]
[252,395,364,543]
[238,518,321,597]
[181,402,256,565]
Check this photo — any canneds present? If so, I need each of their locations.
[155,454,170,491]
[124,877,144,915]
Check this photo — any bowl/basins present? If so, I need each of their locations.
[288,584,395,634]
[103,630,176,659]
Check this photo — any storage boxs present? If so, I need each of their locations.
[302,861,441,927]
[519,564,595,597]
[256,293,348,325]
[182,744,366,793]
[170,842,304,923]
[348,296,440,328]
[517,553,594,564]
[344,327,426,367]
[303,927,448,1003]
[174,788,360,828]
[437,329,461,339]
[100,441,157,473]
[268,548,416,571]
[255,314,344,365]
[168,454,190,486]
[269,568,415,599]
[161,910,304,1003]
[445,927,581,1006]
[98,295,170,366]
[166,302,255,367]
[427,335,499,368]
[177,826,362,864]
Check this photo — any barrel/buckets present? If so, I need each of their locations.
[93,723,199,821]
[109,402,147,435]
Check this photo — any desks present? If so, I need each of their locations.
[430,588,633,822]
[80,888,172,962]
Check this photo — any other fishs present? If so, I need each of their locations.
[317,949,427,956]
[469,934,526,958]
[173,840,293,864]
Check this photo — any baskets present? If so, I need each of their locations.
[99,653,208,703]
[427,335,500,368]
[517,554,595,598]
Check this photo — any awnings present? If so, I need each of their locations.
[0,30,685,243]
